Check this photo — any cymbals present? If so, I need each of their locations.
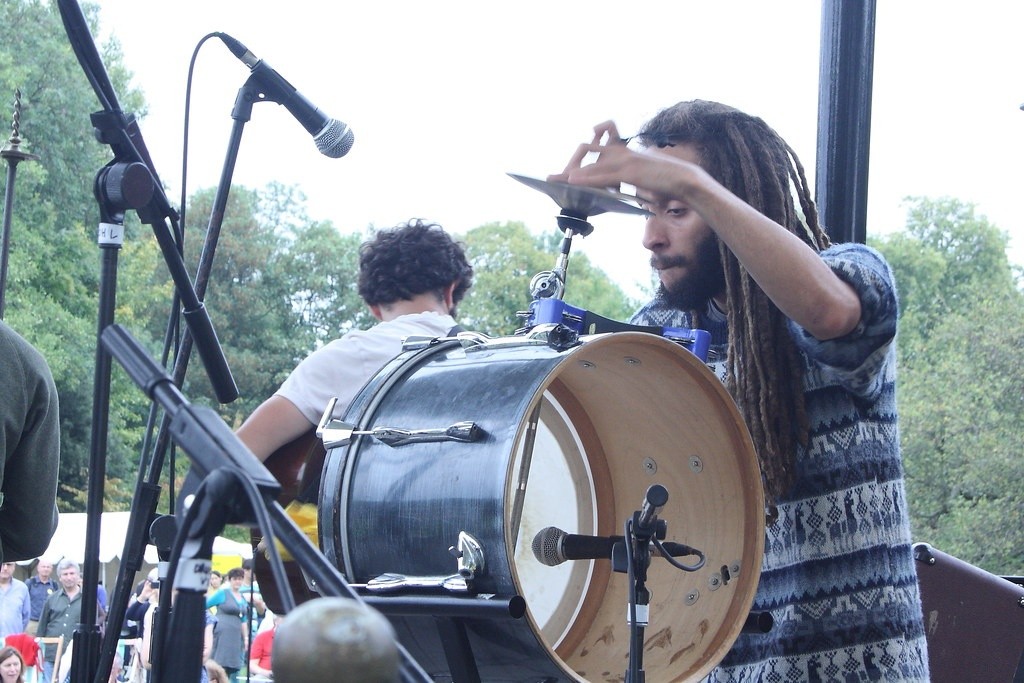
[503,168,656,219]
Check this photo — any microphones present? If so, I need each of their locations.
[531,526,693,567]
[218,32,354,158]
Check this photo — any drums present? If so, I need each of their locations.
[319,324,766,681]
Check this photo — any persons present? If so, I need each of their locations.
[0,559,281,683]
[547,99,932,683]
[235,223,473,462]
[0,321,62,563]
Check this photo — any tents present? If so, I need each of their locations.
[14,510,253,588]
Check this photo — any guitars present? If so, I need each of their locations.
[248,424,328,616]
